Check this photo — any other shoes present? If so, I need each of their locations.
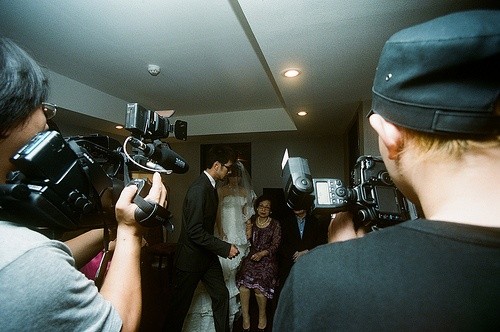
[258,324,266,332]
[242,323,250,332]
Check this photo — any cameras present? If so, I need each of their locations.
[281,148,412,234]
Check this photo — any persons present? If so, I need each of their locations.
[162,145,240,332]
[235,195,282,332]
[271,11,500,332]
[0,37,168,332]
[182,162,258,332]
[272,210,325,315]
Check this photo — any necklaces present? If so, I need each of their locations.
[227,184,241,193]
[257,216,270,225]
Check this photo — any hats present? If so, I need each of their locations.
[364,10,500,136]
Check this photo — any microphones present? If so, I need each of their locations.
[127,137,189,174]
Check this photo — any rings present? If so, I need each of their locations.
[331,214,337,219]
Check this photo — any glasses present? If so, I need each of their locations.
[34,101,58,119]
[258,205,271,211]
[221,164,232,170]
